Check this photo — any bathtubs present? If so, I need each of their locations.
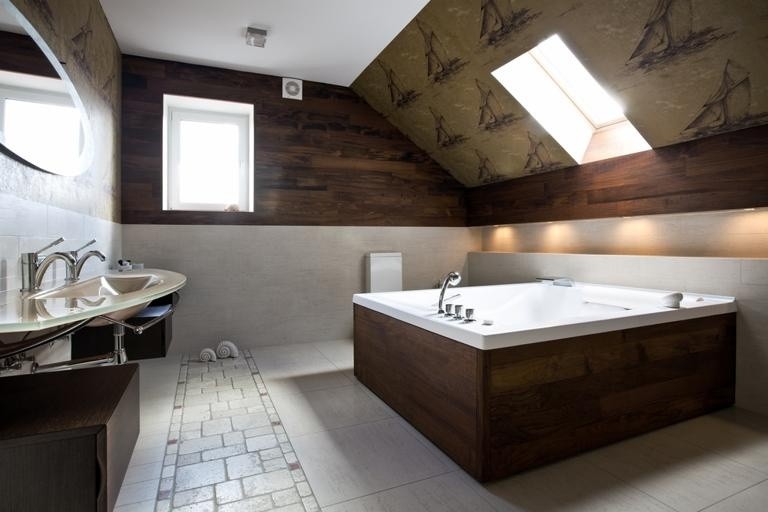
[352,282,738,489]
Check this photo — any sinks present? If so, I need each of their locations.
[27,269,187,327]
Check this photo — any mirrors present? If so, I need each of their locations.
[0,1,95,177]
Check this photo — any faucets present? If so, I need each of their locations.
[434,291,463,309]
[79,297,106,306]
[35,300,57,318]
[36,253,76,287]
[75,251,106,283]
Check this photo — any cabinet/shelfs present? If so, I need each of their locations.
[1,361,145,512]
[71,294,179,368]
